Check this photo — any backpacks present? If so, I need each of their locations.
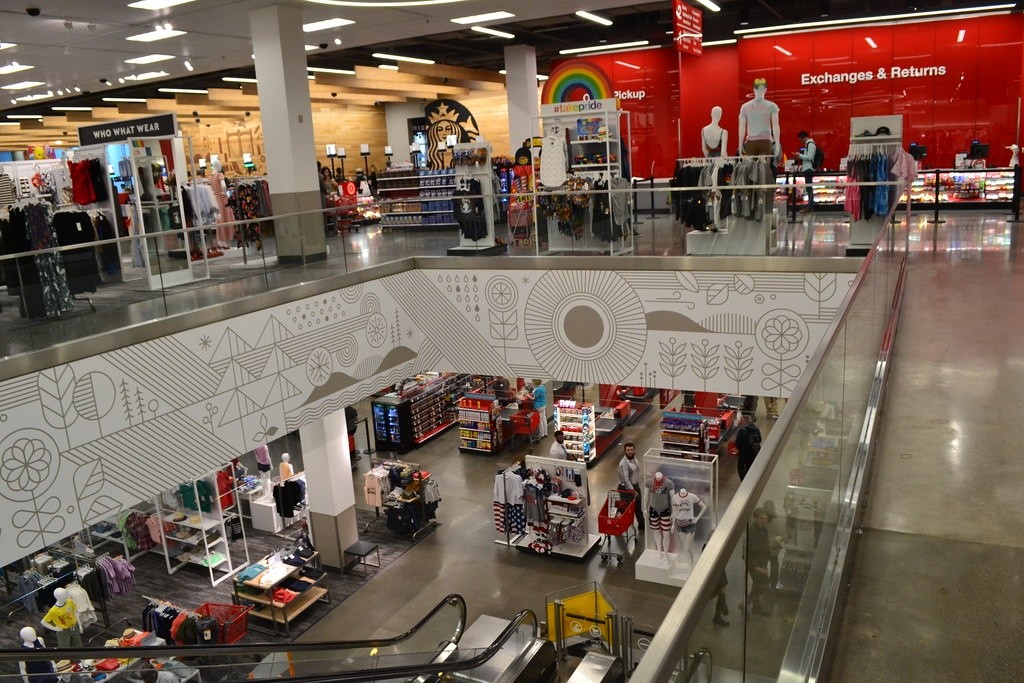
[742,426,762,452]
[806,142,824,169]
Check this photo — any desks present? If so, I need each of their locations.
[250,472,310,533]
[58,629,203,683]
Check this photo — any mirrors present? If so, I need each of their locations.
[128,134,194,292]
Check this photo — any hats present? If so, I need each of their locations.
[173,512,187,522]
[121,629,137,641]
[875,126,891,135]
[56,660,76,673]
[188,515,202,524]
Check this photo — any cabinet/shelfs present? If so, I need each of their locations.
[570,136,620,168]
[553,400,597,471]
[230,545,332,639]
[774,165,1020,213]
[90,462,250,587]
[376,173,460,234]
[371,370,505,456]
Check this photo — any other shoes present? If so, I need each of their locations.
[801,208,812,213]
[751,607,770,615]
[738,602,750,621]
[720,602,729,615]
[713,617,731,626]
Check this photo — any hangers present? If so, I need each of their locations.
[845,142,914,162]
[362,459,439,489]
[17,505,225,628]
[668,155,780,178]
[493,461,586,528]
[274,469,306,489]
[451,166,480,200]
[0,148,110,229]
[539,124,629,189]
[179,174,271,195]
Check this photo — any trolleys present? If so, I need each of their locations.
[598,490,640,564]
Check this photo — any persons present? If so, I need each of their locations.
[492,376,510,390]
[140,664,179,683]
[317,161,367,236]
[209,161,234,249]
[523,379,548,437]
[549,431,575,461]
[702,500,787,626]
[19,587,86,683]
[792,131,815,213]
[735,394,778,482]
[256,444,294,495]
[701,77,780,155]
[617,443,645,530]
[645,472,707,572]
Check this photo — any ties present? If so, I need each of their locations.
[562,445,567,454]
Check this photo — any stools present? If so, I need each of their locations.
[344,540,381,578]
